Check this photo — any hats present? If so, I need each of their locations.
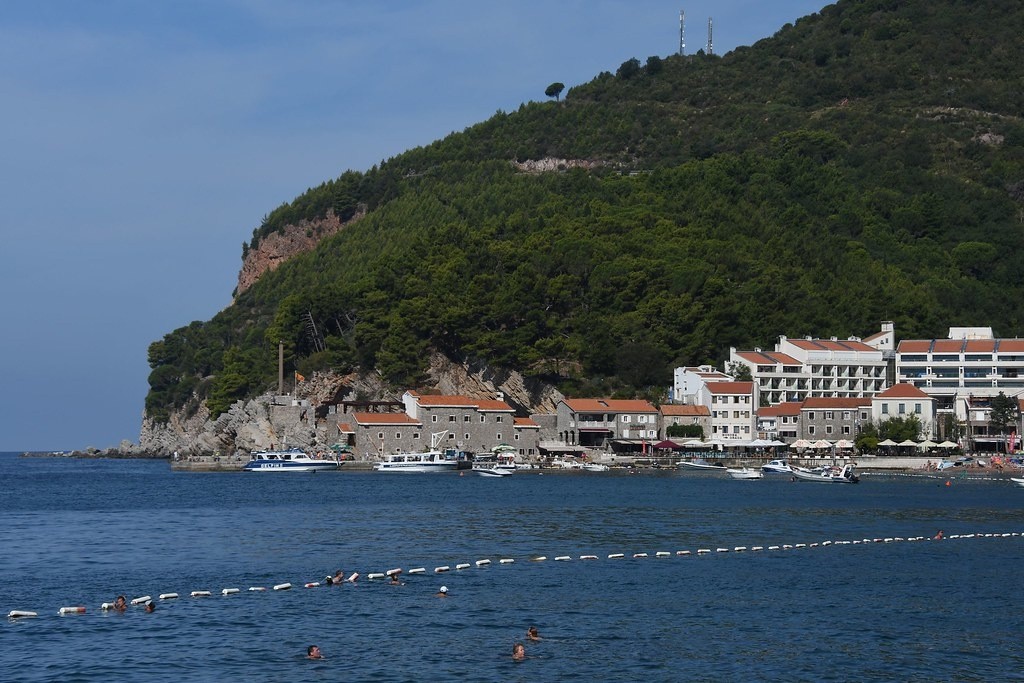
[440,586,448,593]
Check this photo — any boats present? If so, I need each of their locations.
[675,461,728,470]
[792,461,860,483]
[725,468,765,480]
[762,460,794,473]
[582,464,611,472]
[1009,477,1024,482]
[240,446,346,471]
[470,468,514,477]
[376,451,458,472]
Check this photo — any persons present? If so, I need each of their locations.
[512,643,536,660]
[432,585,449,598]
[932,529,944,540]
[304,645,325,660]
[145,600,156,613]
[113,596,127,611]
[524,627,543,641]
[391,574,401,584]
[320,570,343,590]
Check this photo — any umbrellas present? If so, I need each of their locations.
[492,442,517,453]
[790,438,855,456]
[682,438,788,458]
[876,439,959,456]
[654,440,681,449]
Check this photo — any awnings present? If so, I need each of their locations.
[614,439,662,445]
